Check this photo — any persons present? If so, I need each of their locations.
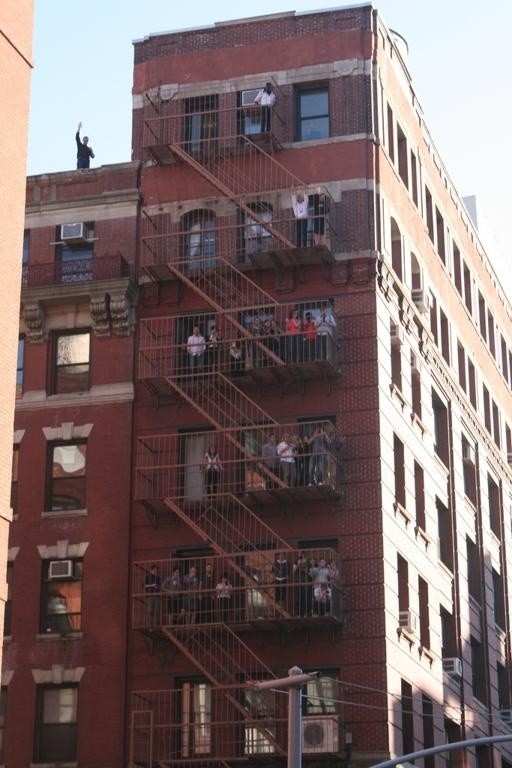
[199,444,226,498]
[242,305,341,366]
[229,338,242,370]
[73,119,96,172]
[313,184,331,247]
[203,322,221,374]
[291,189,313,250]
[237,427,336,494]
[185,323,207,373]
[253,77,279,135]
[141,551,342,627]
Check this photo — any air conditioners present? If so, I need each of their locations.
[463,446,476,464]
[410,289,430,312]
[442,657,462,678]
[60,222,88,241]
[293,715,342,757]
[241,88,264,107]
[399,610,416,633]
[48,559,73,578]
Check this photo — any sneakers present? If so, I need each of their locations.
[256,613,334,618]
[295,613,334,617]
[307,481,325,488]
